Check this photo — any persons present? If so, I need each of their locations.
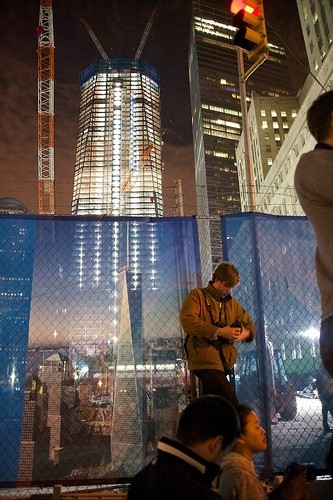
[294,92,333,379]
[271,463,310,500]
[316,360,333,432]
[219,405,272,500]
[180,262,257,405]
[129,394,239,500]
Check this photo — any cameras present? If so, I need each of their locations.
[207,321,240,347]
